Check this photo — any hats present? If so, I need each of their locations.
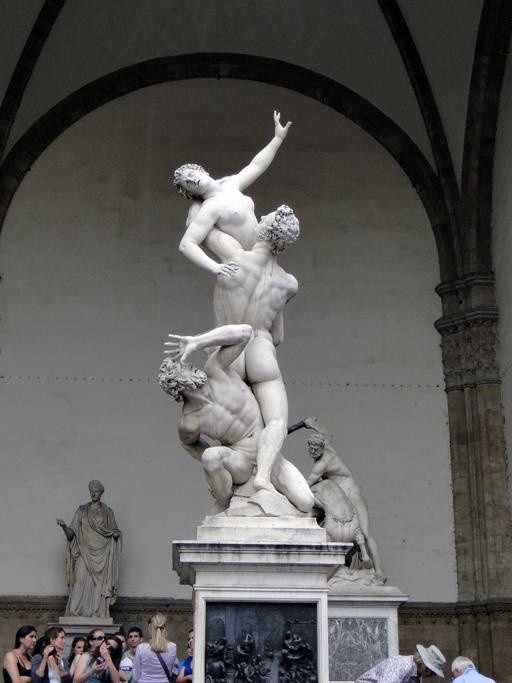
[416,640,447,679]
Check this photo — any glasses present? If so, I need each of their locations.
[90,636,106,640]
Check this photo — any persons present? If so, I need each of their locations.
[302,406,391,586]
[449,655,496,682]
[4,611,193,682]
[184,169,298,489]
[356,637,445,682]
[157,324,315,513]
[56,481,124,616]
[173,110,293,352]
[311,477,369,572]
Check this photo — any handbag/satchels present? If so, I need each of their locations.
[169,673,178,682]
